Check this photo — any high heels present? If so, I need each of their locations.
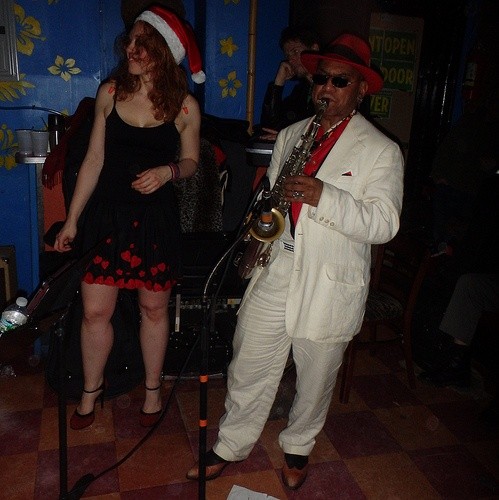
[70,374,109,430]
[140,380,163,428]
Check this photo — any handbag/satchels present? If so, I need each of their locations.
[44,292,146,400]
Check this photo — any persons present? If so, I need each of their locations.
[185,34,403,487]
[54,6,205,429]
[262,26,322,132]
[418,98,499,385]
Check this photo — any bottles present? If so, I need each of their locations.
[0,297,28,338]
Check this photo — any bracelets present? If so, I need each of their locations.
[168,161,180,182]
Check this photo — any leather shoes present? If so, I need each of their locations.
[410,332,472,389]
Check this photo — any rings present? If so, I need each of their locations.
[293,192,304,197]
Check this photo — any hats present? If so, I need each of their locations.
[133,4,207,84]
[300,33,385,94]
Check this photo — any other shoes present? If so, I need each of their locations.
[468,399,498,433]
[281,453,309,490]
[186,452,242,480]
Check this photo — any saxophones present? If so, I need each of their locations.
[233,97,331,280]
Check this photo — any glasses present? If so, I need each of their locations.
[311,73,364,89]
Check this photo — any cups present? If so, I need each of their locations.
[15,131,32,154]
[31,132,49,156]
